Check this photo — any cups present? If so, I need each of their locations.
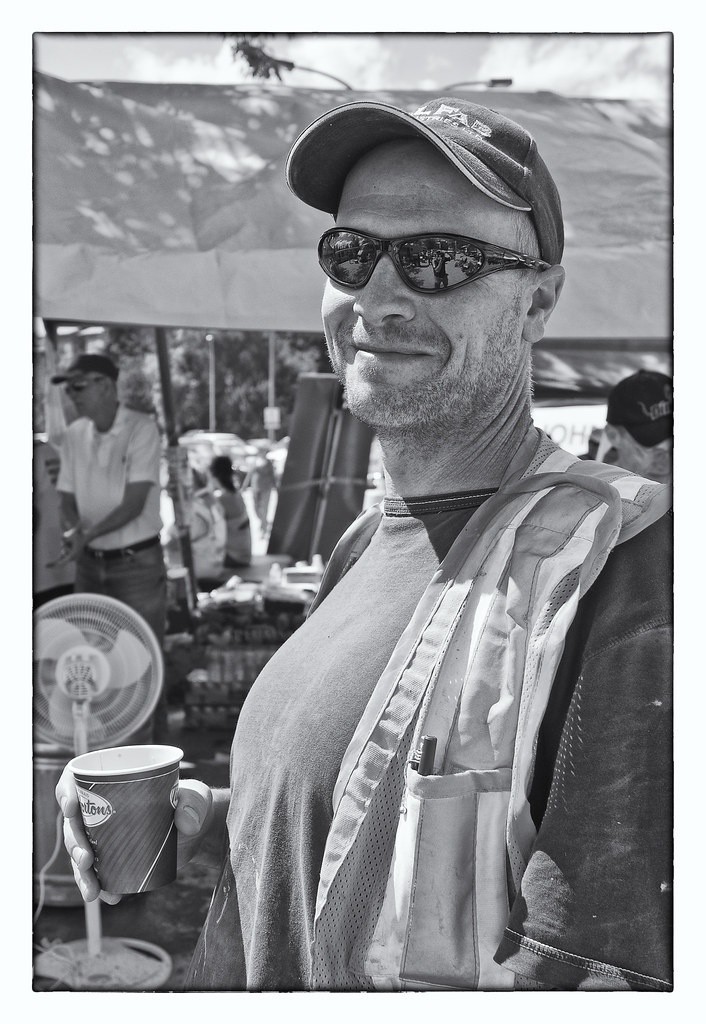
[69,745,183,896]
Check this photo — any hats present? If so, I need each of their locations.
[606,368,674,447]
[284,97,564,266]
[51,354,119,384]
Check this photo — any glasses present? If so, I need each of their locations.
[65,376,108,393]
[317,226,553,295]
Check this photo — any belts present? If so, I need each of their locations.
[84,535,161,561]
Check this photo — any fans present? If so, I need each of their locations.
[33,590,174,992]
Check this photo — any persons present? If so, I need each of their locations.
[51,94,673,992]
[238,446,282,536]
[50,353,168,745]
[605,367,673,484]
[202,455,252,571]
[158,466,228,592]
[32,432,78,610]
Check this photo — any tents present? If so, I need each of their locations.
[33,84,673,573]
